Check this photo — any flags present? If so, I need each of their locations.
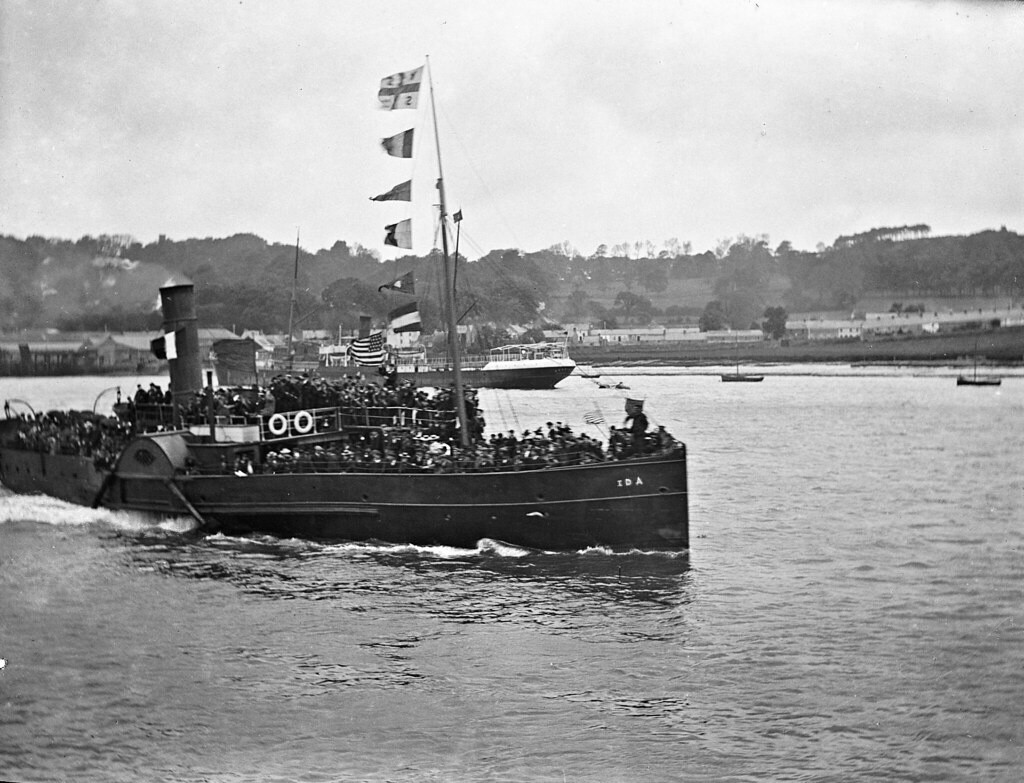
[387,301,423,333]
[453,210,463,224]
[378,65,424,111]
[380,128,414,158]
[378,271,415,295]
[384,219,413,249]
[584,411,603,425]
[368,178,411,202]
[150,326,188,361]
[350,332,385,367]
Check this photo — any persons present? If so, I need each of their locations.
[18,363,675,473]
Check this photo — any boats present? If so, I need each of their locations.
[1,53,693,560]
[956,373,1002,386]
[258,342,576,390]
[721,373,764,382]
